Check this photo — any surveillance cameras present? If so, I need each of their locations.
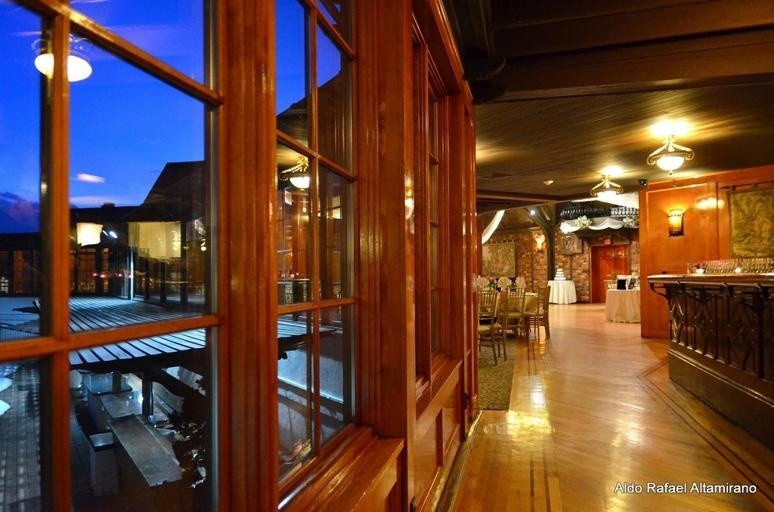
[640,179,648,186]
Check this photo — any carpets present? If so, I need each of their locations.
[474,329,519,413]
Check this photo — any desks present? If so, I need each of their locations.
[604,283,641,324]
[544,278,578,305]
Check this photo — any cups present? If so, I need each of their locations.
[685,257,774,272]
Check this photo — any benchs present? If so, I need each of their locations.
[1,295,338,509]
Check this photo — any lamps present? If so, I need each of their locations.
[645,132,696,176]
[28,26,95,86]
[588,173,624,202]
[276,153,314,191]
[665,208,685,237]
[535,240,546,257]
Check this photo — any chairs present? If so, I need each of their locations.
[476,269,553,365]
